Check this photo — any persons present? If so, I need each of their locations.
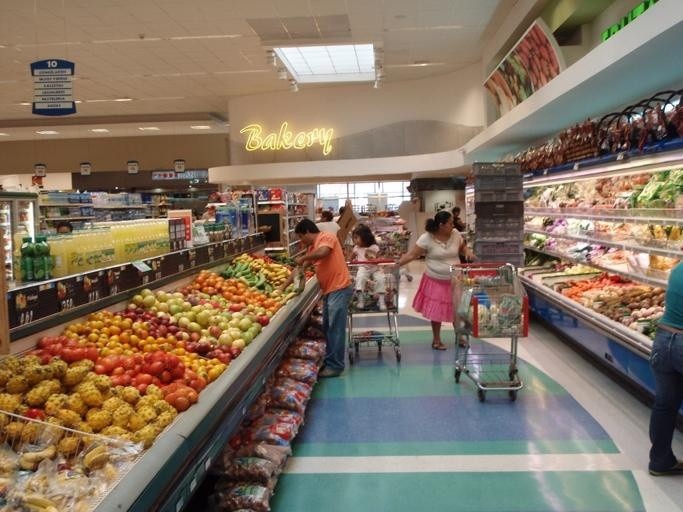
[315,206,346,237]
[201,205,216,220]
[346,224,389,311]
[384,212,478,351]
[649,262,683,477]
[449,205,467,232]
[278,219,353,377]
[56,222,73,233]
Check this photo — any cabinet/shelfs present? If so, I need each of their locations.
[254,192,315,263]
[0,256,320,512]
[38,202,149,235]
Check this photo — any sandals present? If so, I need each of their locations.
[459,340,470,348]
[432,342,447,350]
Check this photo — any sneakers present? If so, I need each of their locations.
[648,460,683,477]
[376,301,387,311]
[356,301,365,309]
[318,368,340,377]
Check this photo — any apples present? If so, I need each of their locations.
[114,269,283,365]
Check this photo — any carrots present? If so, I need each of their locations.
[561,278,606,302]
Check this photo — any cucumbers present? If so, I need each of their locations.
[222,253,290,295]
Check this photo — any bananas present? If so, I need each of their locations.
[0,437,117,512]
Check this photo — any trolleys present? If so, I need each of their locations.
[345,258,402,362]
[448,261,530,403]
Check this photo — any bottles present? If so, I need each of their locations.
[91,217,170,265]
[12,224,117,282]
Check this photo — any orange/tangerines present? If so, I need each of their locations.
[63,310,227,382]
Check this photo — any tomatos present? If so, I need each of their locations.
[30,335,206,412]
[25,409,45,422]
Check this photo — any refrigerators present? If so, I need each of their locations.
[0,191,40,283]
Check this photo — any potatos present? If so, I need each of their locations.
[599,287,667,322]
